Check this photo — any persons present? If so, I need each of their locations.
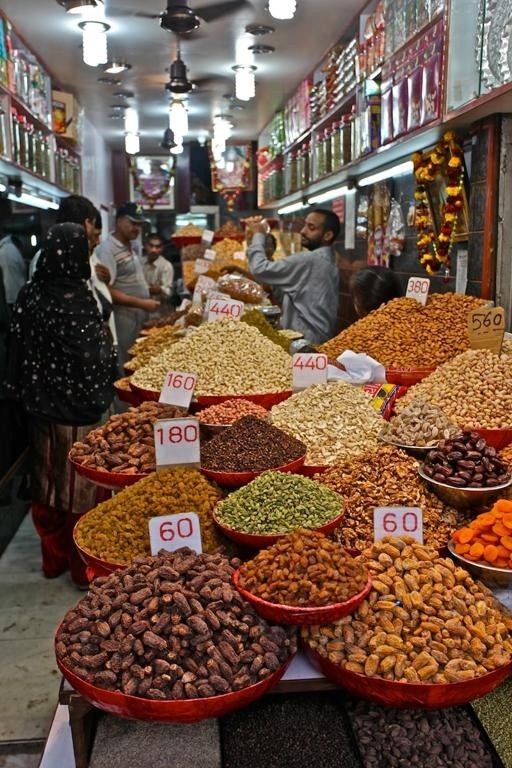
[350,265,401,318]
[1,194,175,589]
[246,209,341,346]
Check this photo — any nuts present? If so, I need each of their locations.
[55,276,512,768]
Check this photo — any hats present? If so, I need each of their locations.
[117,202,151,223]
